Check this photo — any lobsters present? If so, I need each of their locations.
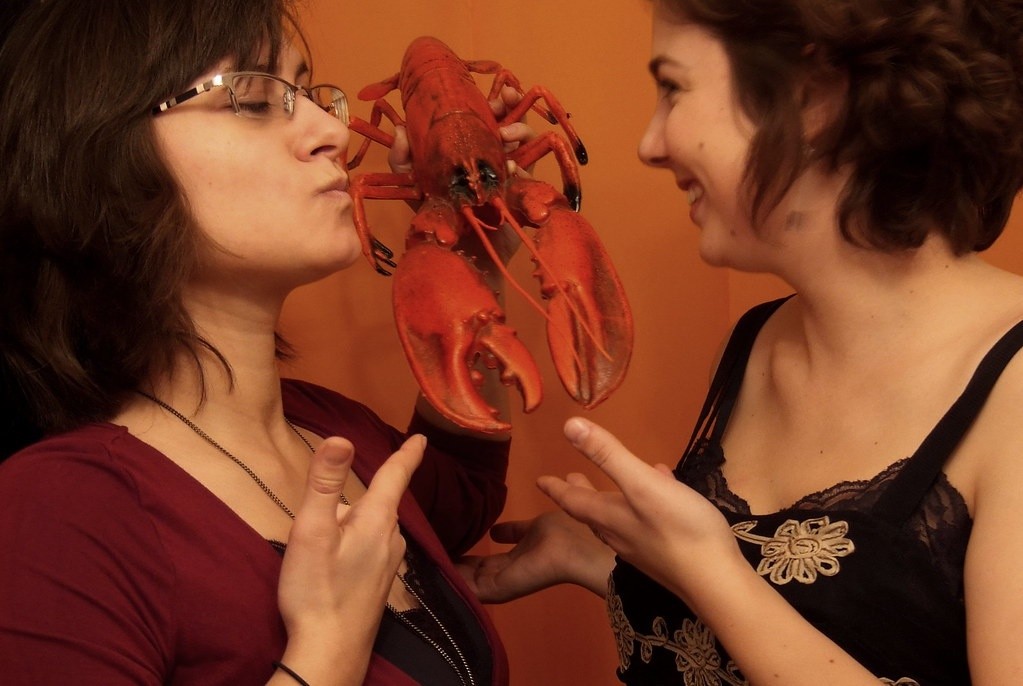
[336,35,635,436]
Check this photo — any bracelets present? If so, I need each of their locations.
[270,658,310,686]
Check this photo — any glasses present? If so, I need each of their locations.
[151,71,350,128]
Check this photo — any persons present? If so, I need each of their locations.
[457,1,1023,686]
[0,0,536,686]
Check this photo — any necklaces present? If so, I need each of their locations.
[134,388,477,686]
